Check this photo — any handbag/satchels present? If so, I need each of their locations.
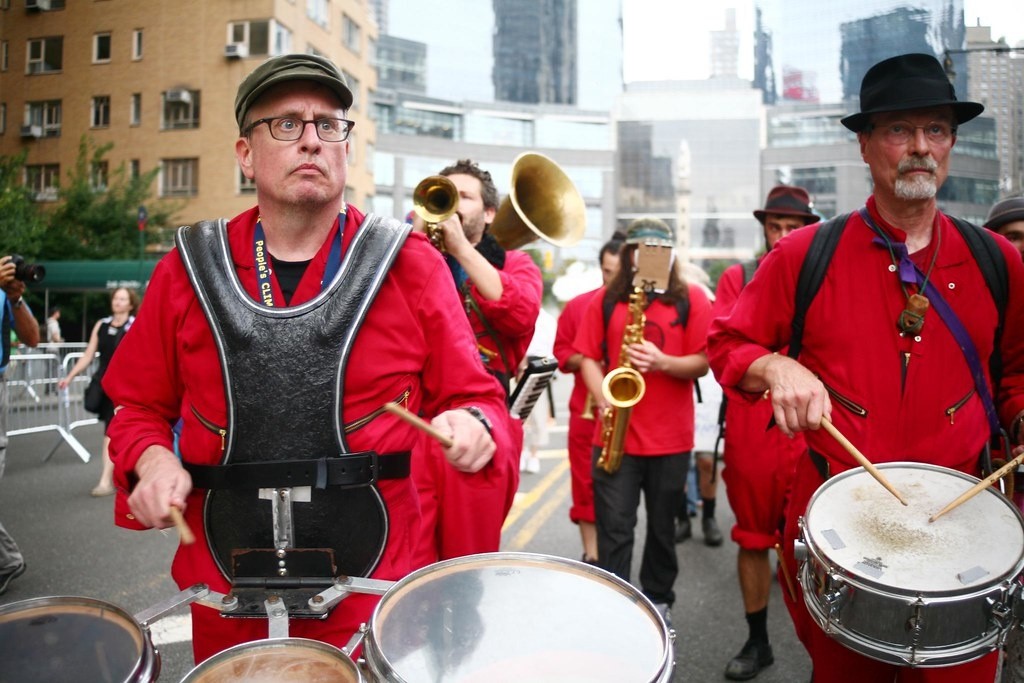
[84,372,100,413]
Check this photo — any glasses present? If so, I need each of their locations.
[878,119,953,145]
[244,116,355,142]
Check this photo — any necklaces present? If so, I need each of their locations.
[865,206,941,340]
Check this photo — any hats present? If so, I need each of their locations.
[841,54,984,133]
[235,52,353,131]
[624,217,672,244]
[753,186,820,225]
[983,198,1024,228]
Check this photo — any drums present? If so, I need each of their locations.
[364,552,673,683]
[800,463,1024,670]
[2,595,165,681]
[174,638,357,681]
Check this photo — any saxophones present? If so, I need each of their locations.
[596,286,650,474]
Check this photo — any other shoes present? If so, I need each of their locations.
[655,603,671,628]
[91,486,117,497]
[703,516,723,547]
[724,642,775,681]
[675,518,691,541]
[0,563,25,594]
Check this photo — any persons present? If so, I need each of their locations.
[982,198,1024,266]
[572,218,713,633]
[553,230,628,567]
[59,288,138,496]
[0,256,40,595]
[707,54,1024,682]
[673,370,725,545]
[103,55,506,670]
[43,306,66,395]
[405,160,543,559]
[705,186,822,683]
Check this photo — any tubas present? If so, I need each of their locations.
[480,152,590,251]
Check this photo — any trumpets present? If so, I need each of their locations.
[414,172,462,254]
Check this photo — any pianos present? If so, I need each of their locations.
[507,356,560,428]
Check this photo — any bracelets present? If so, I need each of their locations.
[761,352,778,398]
[1013,416,1024,446]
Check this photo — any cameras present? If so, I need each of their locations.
[6,255,45,282]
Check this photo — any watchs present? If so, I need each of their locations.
[10,296,23,309]
[466,407,493,430]
